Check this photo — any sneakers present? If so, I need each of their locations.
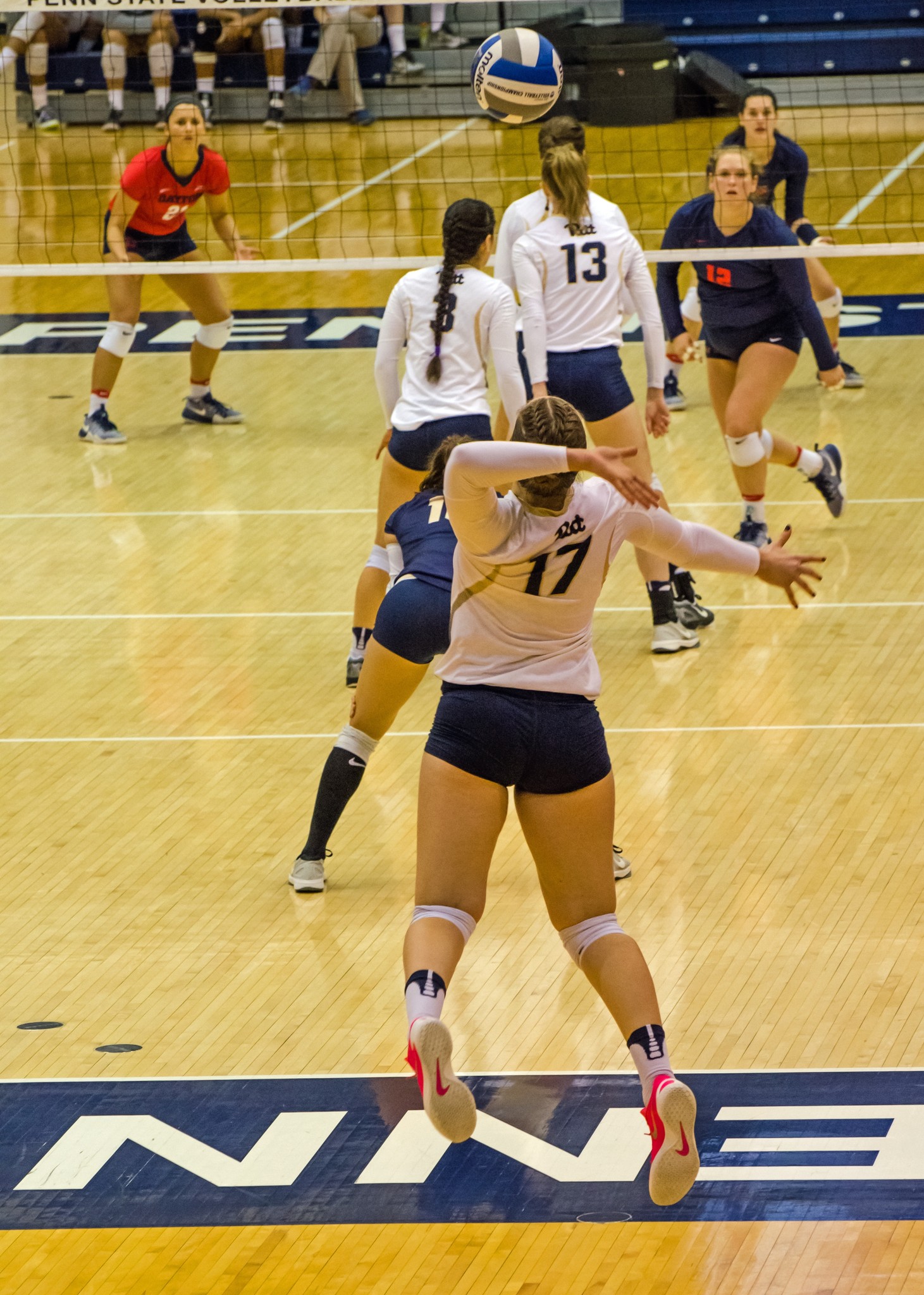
[180,391,244,424]
[804,442,847,519]
[733,515,773,550]
[78,402,126,444]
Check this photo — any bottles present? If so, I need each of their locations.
[419,21,430,49]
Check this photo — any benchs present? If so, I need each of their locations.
[620,0,924,75]
[16,9,394,88]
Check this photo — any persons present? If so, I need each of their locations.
[512,147,702,656]
[77,94,270,445]
[346,197,529,685]
[286,432,632,893]
[664,88,864,412]
[656,145,846,548]
[403,396,825,1207]
[0,3,470,131]
[494,116,715,628]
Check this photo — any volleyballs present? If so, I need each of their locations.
[470,27,563,124]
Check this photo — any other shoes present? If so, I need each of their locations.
[356,110,373,126]
[287,75,312,101]
[203,110,214,129]
[673,595,715,628]
[640,1074,700,1206]
[263,106,284,129]
[102,108,123,131]
[815,351,866,386]
[614,845,630,881]
[34,103,61,130]
[345,656,365,686]
[288,846,333,893]
[664,369,688,411]
[155,109,168,131]
[428,31,469,49]
[394,56,424,74]
[651,617,701,653]
[405,1016,476,1143]
